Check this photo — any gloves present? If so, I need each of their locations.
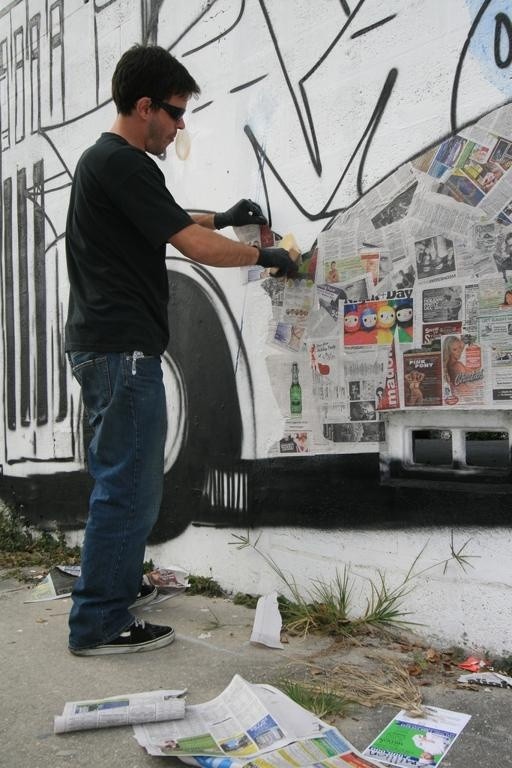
[212,198,267,230]
[251,244,299,280]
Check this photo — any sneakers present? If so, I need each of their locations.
[128,585,160,609]
[70,615,175,657]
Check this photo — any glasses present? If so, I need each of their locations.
[161,100,186,122]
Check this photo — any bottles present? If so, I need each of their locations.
[289,363,305,417]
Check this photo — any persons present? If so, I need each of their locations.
[443,337,476,396]
[327,261,338,283]
[502,233,512,270]
[405,370,425,406]
[500,291,512,306]
[376,387,383,403]
[413,732,449,765]
[64,45,298,657]
[349,383,361,400]
[298,436,308,453]
